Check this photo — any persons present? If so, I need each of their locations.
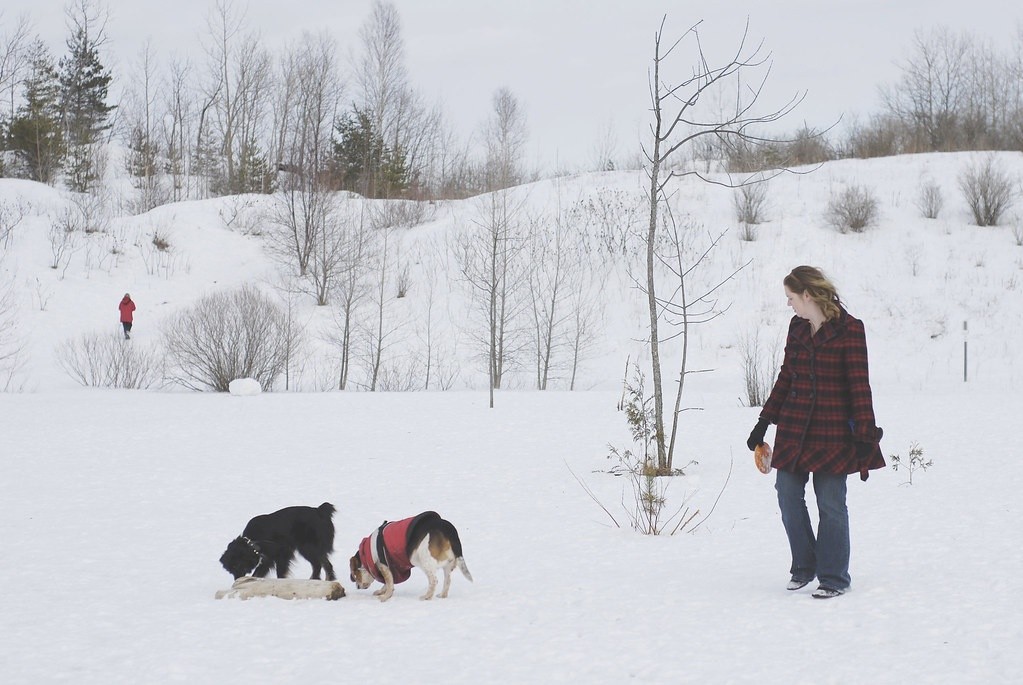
[747,266,886,598]
[119,293,136,339]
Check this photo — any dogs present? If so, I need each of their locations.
[349,511,472,603]
[220,502,336,581]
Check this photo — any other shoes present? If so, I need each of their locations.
[787,579,809,590]
[126,329,130,335]
[812,585,851,596]
[124,332,130,339]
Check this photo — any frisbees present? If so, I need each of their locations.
[754,442,773,473]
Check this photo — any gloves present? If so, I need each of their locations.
[854,443,875,466]
[746,418,771,451]
